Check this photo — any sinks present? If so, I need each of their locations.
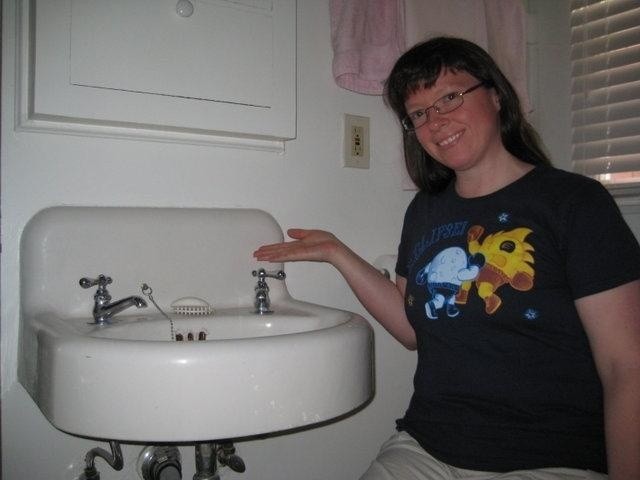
[17,303,375,443]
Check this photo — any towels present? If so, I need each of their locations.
[328,0,534,118]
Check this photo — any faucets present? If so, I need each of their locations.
[79,275,148,322]
[252,269,287,313]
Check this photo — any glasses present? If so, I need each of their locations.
[401,83,485,131]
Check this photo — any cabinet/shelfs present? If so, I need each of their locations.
[12,0,296,153]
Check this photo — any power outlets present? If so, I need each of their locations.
[338,112,370,168]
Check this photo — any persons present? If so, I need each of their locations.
[252,35,640,480]
